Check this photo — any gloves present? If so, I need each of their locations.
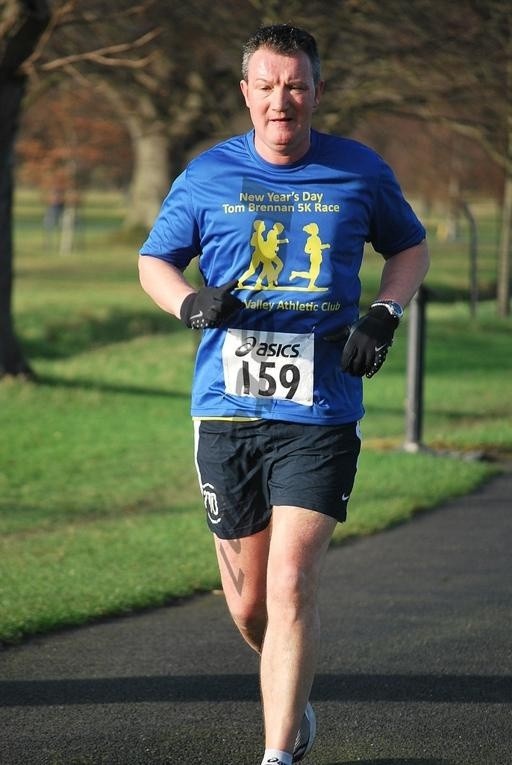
[178,279,243,331]
[323,308,398,378]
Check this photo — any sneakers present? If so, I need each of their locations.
[291,703,316,762]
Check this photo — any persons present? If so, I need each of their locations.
[255,222,290,289]
[289,221,331,291]
[134,19,433,763]
[236,219,275,290]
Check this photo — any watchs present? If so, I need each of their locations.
[369,298,403,318]
